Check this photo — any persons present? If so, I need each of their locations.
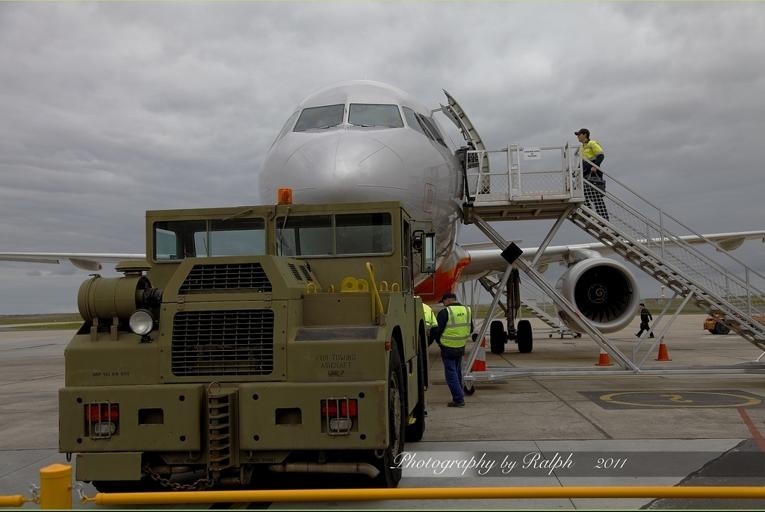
[635,303,655,338]
[421,293,474,407]
[575,128,609,221]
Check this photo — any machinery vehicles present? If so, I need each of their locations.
[56,188,429,503]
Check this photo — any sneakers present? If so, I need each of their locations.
[448,400,465,407]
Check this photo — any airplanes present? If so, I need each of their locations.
[0,78,765,355]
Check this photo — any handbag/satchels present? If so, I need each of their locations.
[640,322,649,330]
[583,177,606,197]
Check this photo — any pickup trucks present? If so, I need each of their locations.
[703,303,765,335]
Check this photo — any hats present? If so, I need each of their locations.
[575,128,590,135]
[438,293,456,303]
